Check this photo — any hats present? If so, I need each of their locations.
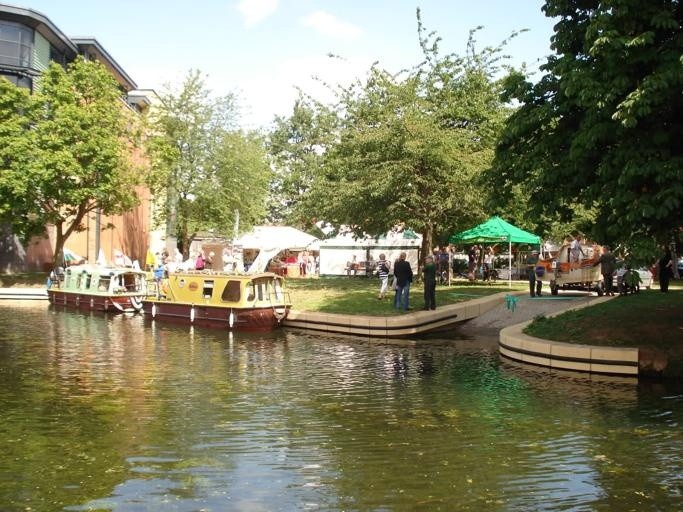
[531,250,537,254]
[423,255,432,259]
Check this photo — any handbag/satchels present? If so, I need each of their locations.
[381,264,388,273]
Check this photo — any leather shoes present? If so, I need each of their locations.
[421,307,428,310]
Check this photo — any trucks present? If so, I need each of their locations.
[550,244,604,297]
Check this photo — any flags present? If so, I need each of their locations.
[61,246,81,261]
[97,248,140,269]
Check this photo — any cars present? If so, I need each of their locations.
[601,265,654,292]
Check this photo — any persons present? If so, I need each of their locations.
[346,255,357,277]
[369,252,413,310]
[152,247,319,277]
[620,266,634,296]
[482,246,494,282]
[525,250,541,297]
[659,249,672,293]
[421,256,436,310]
[569,234,588,271]
[591,245,617,296]
[467,245,478,281]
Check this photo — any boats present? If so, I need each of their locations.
[46,264,147,312]
[140,269,292,332]
